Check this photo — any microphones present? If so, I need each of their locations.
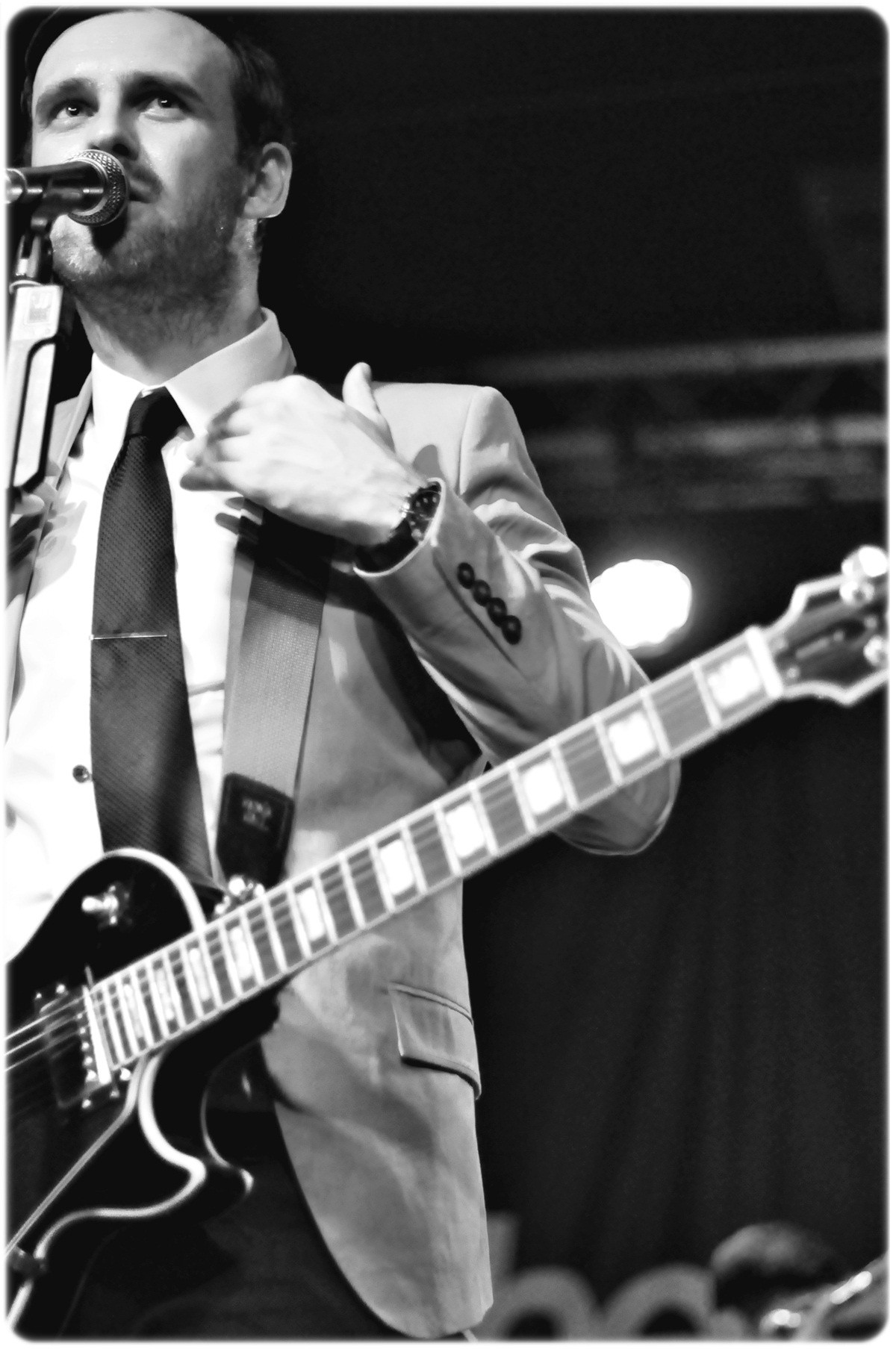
[3,149,130,227]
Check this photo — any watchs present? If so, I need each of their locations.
[355,480,443,574]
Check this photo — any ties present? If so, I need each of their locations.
[92,389,215,892]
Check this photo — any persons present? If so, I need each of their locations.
[4,8,680,1342]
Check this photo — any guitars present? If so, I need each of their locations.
[7,546,889,1341]
